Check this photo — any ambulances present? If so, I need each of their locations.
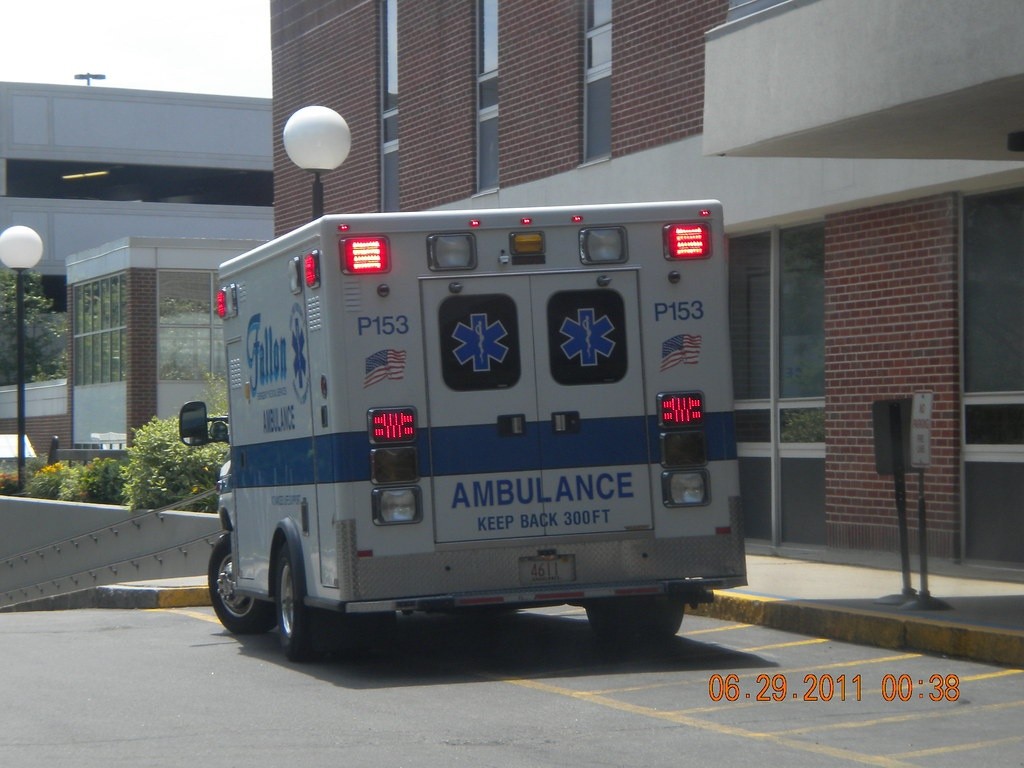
[168,192,752,671]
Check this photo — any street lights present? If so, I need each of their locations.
[273,101,353,227]
[0,218,45,492]
